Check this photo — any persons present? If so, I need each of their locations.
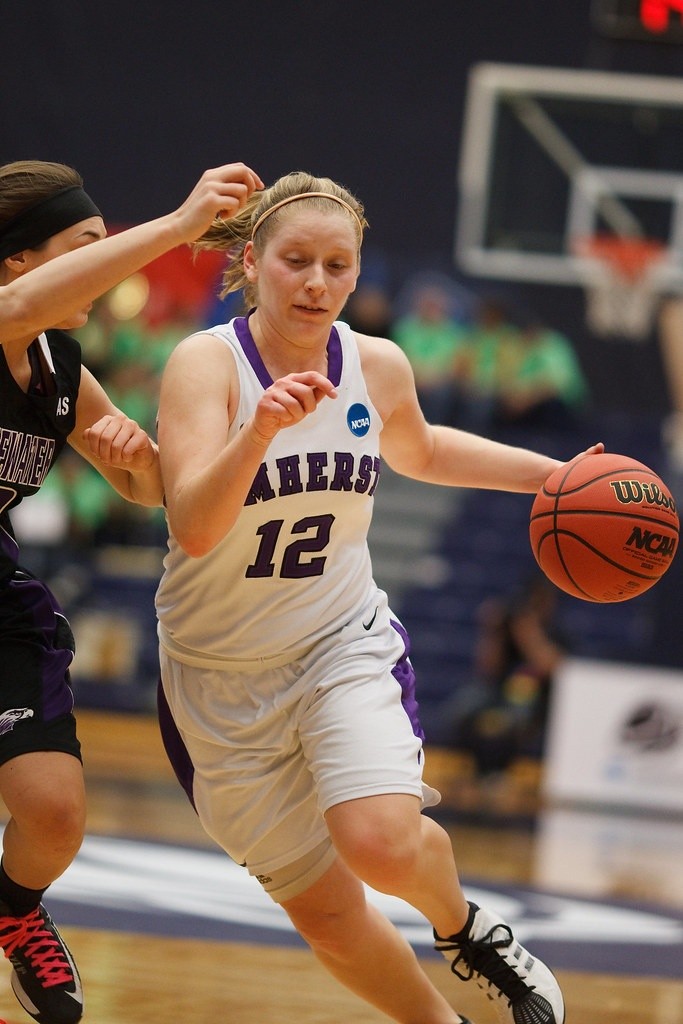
[157,172,606,1023]
[0,251,683,740]
[0,159,265,1023]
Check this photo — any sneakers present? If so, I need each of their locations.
[434,900,566,1024]
[0,899,83,1024]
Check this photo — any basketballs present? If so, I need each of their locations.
[528,453,682,607]
